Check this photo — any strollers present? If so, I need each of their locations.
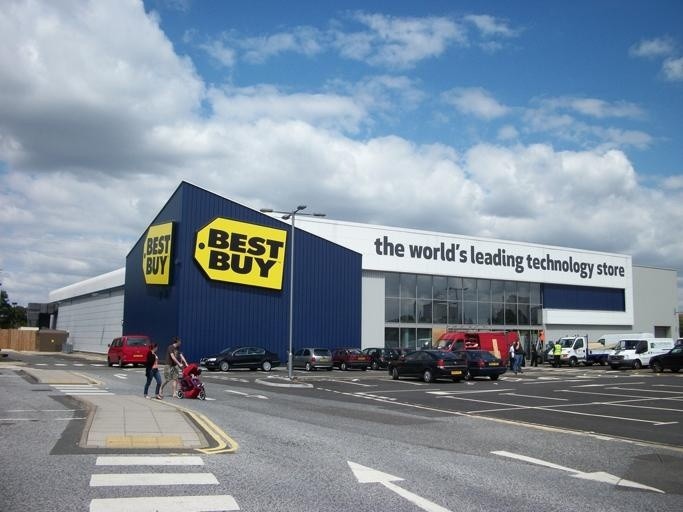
[175,362,206,399]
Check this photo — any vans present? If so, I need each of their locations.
[106,335,152,368]
[285,347,333,371]
[608,337,675,371]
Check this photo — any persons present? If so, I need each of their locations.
[143,343,163,399]
[509,332,562,374]
[159,336,188,399]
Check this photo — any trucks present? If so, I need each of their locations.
[546,335,616,367]
[595,333,655,348]
[433,331,519,368]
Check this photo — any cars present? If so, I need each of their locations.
[649,344,682,374]
[327,347,370,370]
[534,348,553,364]
[452,350,507,380]
[200,344,281,373]
[387,350,467,383]
[362,347,399,370]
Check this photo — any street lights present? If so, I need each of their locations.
[10,302,17,314]
[444,284,468,323]
[257,204,327,380]
